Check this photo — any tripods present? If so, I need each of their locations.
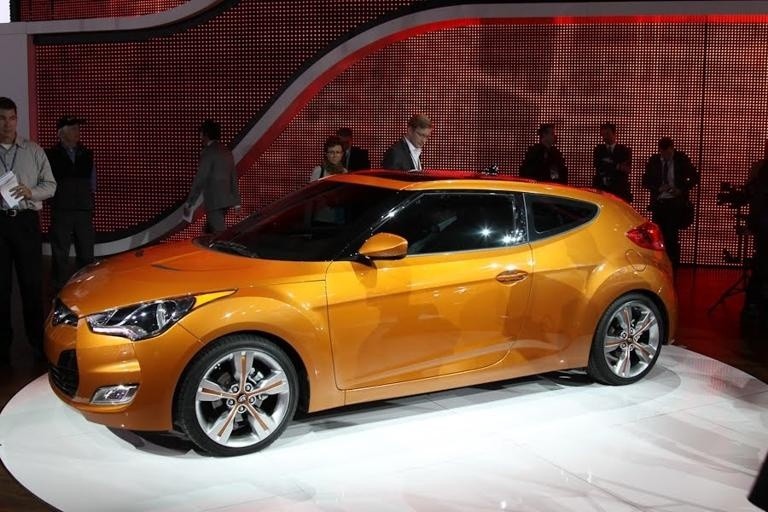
[706,207,751,315]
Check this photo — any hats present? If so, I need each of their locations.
[57,115,86,128]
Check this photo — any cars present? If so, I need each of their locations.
[44,169,686,458]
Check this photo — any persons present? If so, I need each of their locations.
[335,127,371,174]
[0,96,59,380]
[382,113,434,172]
[183,118,238,238]
[519,123,568,186]
[41,115,97,297]
[642,138,697,270]
[591,123,632,206]
[402,197,489,255]
[309,135,348,185]
[730,139,767,363]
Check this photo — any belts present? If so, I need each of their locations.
[0,208,19,218]
